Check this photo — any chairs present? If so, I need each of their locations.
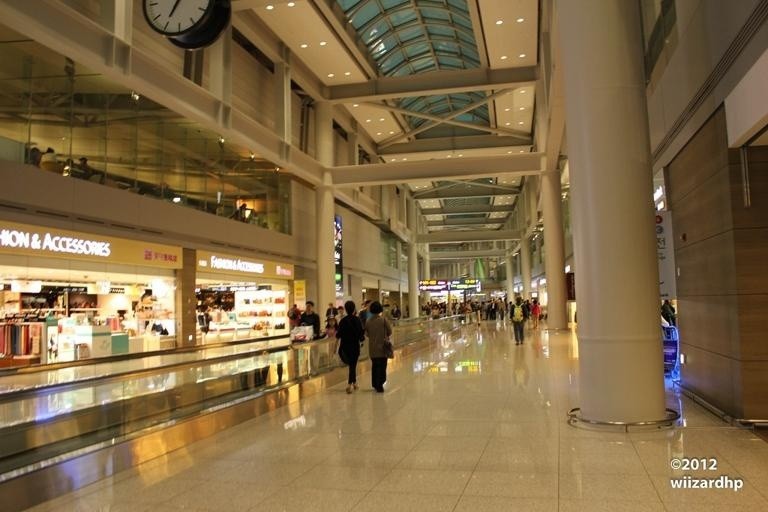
[26,159,104,184]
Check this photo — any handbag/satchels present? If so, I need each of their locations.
[381,317,395,360]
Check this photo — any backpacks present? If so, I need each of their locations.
[511,302,524,324]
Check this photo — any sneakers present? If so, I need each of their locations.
[344,382,360,395]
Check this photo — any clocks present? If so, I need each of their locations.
[143,1,232,50]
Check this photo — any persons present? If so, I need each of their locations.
[287,304,302,334]
[358,299,402,326]
[530,329,543,360]
[364,391,395,498]
[337,391,364,478]
[363,300,393,394]
[513,343,530,393]
[665,300,675,313]
[510,296,528,345]
[661,304,676,327]
[214,200,260,225]
[320,314,341,367]
[334,300,366,394]
[335,306,348,356]
[298,300,322,375]
[326,302,338,318]
[24,145,93,179]
[421,297,543,330]
